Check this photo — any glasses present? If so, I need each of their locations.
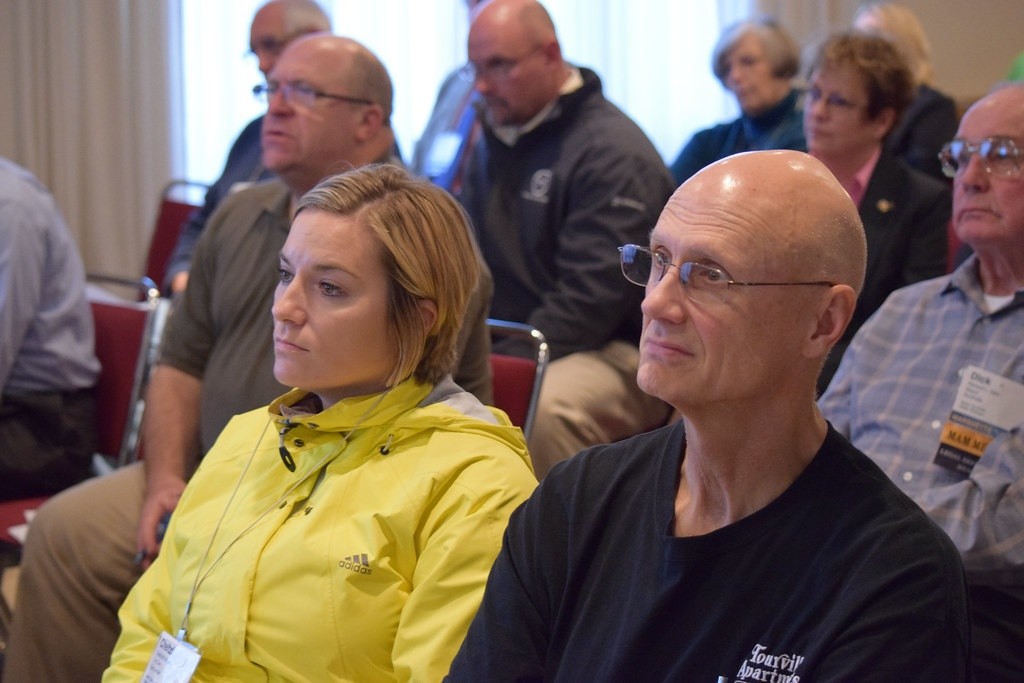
[617,244,836,306]
[252,81,373,106]
[938,137,1024,177]
[806,88,856,111]
[457,47,543,82]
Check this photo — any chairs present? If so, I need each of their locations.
[487,318,549,444]
[0,409,146,548]
[137,179,210,302]
[0,273,160,469]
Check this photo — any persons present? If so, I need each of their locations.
[449,1,673,484]
[791,29,950,401]
[664,17,808,189]
[100,161,537,683]
[2,35,392,682]
[162,1,332,300]
[0,158,104,504]
[854,6,957,182]
[435,150,973,683]
[818,85,1023,683]
